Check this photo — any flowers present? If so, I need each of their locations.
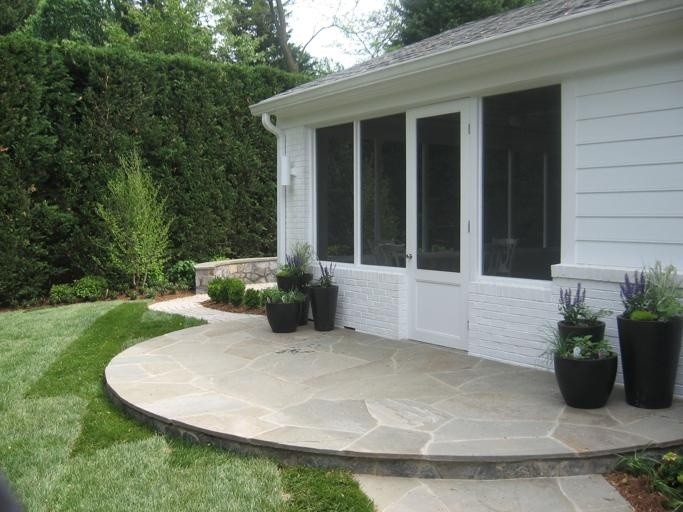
[310,261,336,288]
[558,283,614,327]
[259,287,306,305]
[275,240,312,277]
[539,323,611,368]
[620,261,683,322]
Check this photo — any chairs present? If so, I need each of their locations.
[366,239,520,275]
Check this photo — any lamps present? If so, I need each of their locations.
[281,157,297,186]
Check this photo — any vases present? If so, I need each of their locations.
[557,321,605,343]
[617,314,683,410]
[552,352,618,409]
[266,303,303,333]
[277,276,312,326]
[310,286,338,331]
